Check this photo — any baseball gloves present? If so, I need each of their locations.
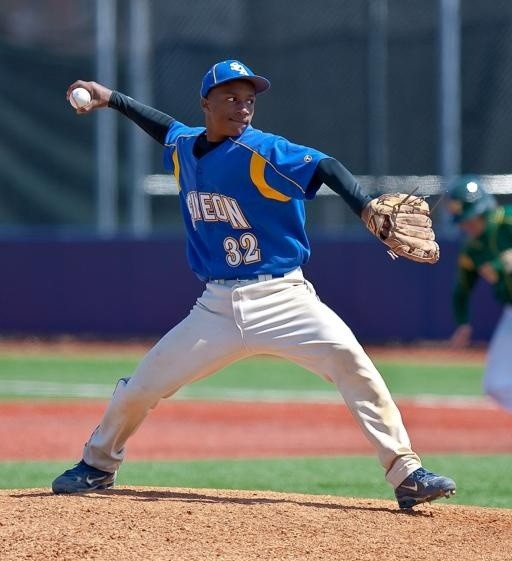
[366,193,440,265]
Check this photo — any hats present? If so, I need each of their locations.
[198,57,272,99]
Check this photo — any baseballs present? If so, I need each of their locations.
[70,87,91,108]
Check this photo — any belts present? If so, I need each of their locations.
[205,271,285,290]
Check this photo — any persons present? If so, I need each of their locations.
[49,58,458,510]
[441,171,512,413]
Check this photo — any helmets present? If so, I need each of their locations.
[446,176,497,228]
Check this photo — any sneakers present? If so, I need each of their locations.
[50,458,118,497]
[394,465,458,509]
